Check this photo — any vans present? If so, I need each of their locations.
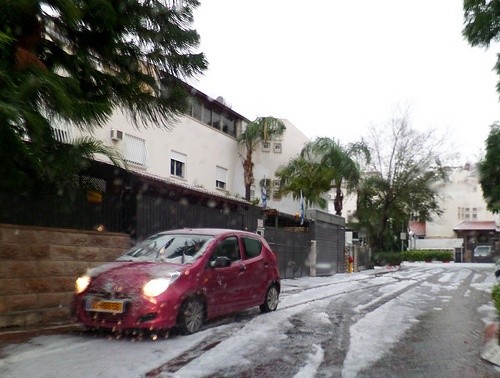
[472,245,495,263]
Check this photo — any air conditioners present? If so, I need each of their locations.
[110,128,124,142]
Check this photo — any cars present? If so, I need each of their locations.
[69,227,281,335]
